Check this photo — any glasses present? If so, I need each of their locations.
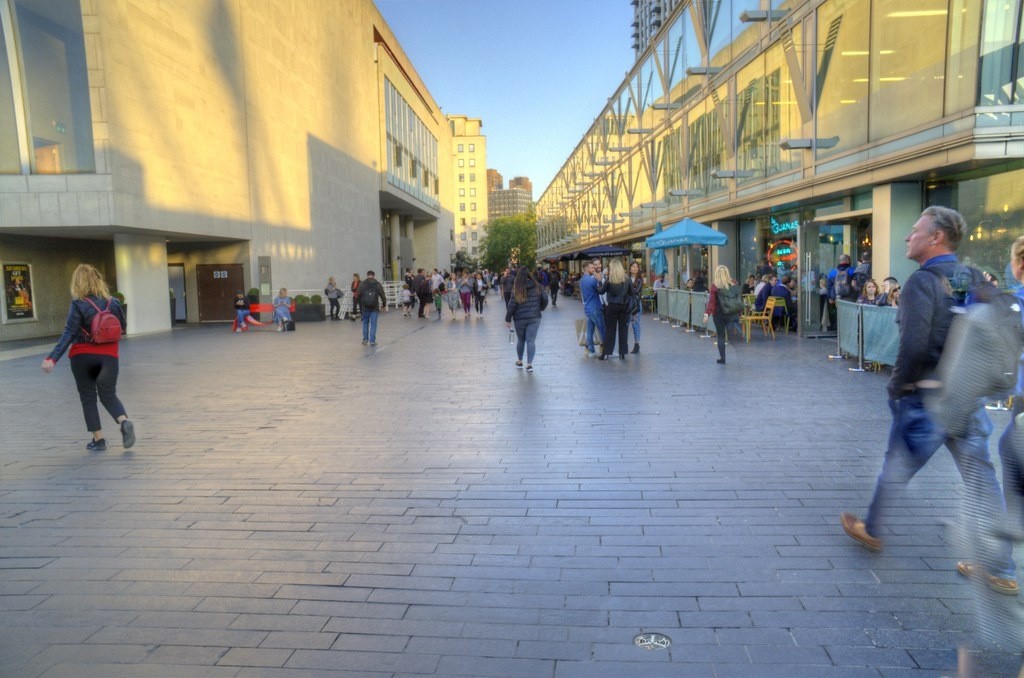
[893,285,901,292]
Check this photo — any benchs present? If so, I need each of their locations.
[232,300,295,332]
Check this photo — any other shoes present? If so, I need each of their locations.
[362,340,367,345]
[419,314,430,319]
[465,314,471,319]
[475,314,483,320]
[717,358,725,364]
[276,327,282,332]
[403,312,411,317]
[599,347,603,353]
[631,343,639,353]
[331,317,342,320]
[584,348,596,357]
[236,327,242,332]
[240,322,246,328]
[371,342,378,346]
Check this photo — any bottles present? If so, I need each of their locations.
[509,329,515,344]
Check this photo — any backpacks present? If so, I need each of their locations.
[717,286,743,315]
[81,297,122,344]
[948,282,1019,401]
[364,279,379,308]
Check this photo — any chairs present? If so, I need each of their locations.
[642,286,656,313]
[735,292,791,344]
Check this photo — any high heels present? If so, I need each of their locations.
[597,354,626,360]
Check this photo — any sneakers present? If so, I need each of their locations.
[121,420,135,448]
[515,361,533,372]
[86,438,106,450]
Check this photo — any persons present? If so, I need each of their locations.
[10,279,32,305]
[840,206,1024,594]
[641,266,709,314]
[272,288,293,332]
[233,289,250,333]
[741,250,902,331]
[351,273,363,321]
[579,258,643,360]
[505,268,548,372]
[325,277,343,320]
[41,264,135,451]
[356,271,386,345]
[703,265,743,364]
[397,265,580,324]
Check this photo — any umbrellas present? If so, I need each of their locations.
[645,217,729,281]
[542,245,630,276]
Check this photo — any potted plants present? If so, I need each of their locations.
[295,294,326,322]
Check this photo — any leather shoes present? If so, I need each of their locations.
[957,562,1018,595]
[840,512,884,553]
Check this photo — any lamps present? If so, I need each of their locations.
[640,204,654,208]
[533,225,609,253]
[619,212,642,217]
[603,219,623,224]
[652,101,668,110]
[533,182,593,225]
[669,189,687,196]
[583,173,604,177]
[628,129,640,134]
[595,162,613,166]
[687,66,706,74]
[738,9,768,21]
[609,147,620,152]
[710,169,733,178]
[779,139,811,150]
